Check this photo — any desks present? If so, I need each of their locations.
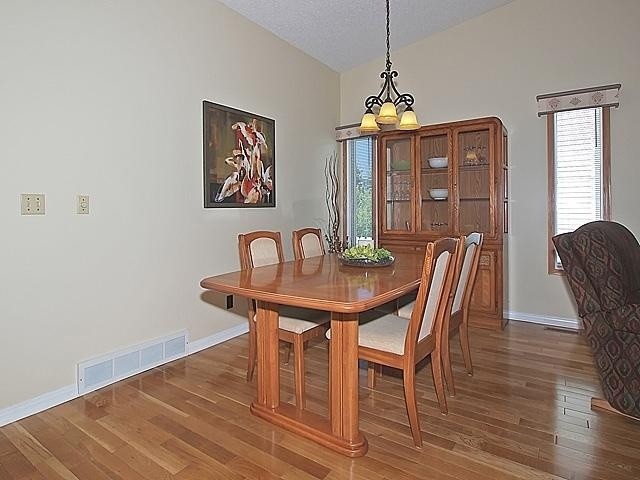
[199,252,437,459]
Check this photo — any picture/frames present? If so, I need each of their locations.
[202,100,276,208]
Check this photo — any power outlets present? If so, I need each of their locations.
[20,194,45,215]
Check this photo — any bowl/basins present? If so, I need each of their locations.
[428,189,448,200]
[389,159,411,170]
[427,157,448,168]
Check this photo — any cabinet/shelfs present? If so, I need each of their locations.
[377,116,509,333]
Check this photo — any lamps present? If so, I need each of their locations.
[357,0,422,131]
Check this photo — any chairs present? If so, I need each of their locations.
[237,231,331,410]
[325,238,461,448]
[283,228,326,365]
[374,232,484,377]
[551,221,639,420]
